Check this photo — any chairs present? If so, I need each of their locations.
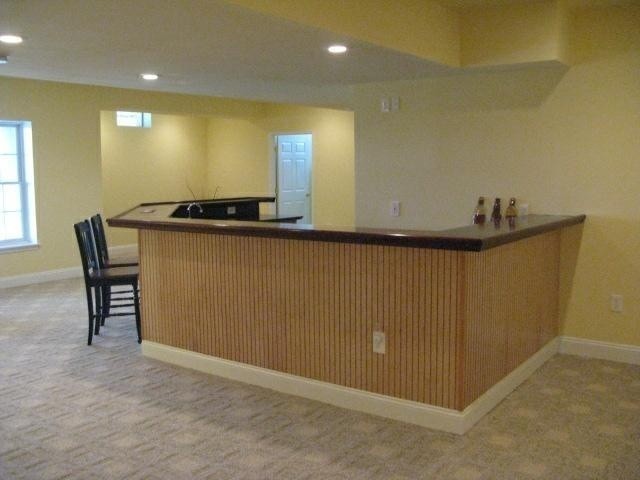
[74,215,141,346]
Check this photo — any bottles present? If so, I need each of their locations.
[473,197,519,222]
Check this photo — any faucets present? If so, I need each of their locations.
[186,202,204,218]
